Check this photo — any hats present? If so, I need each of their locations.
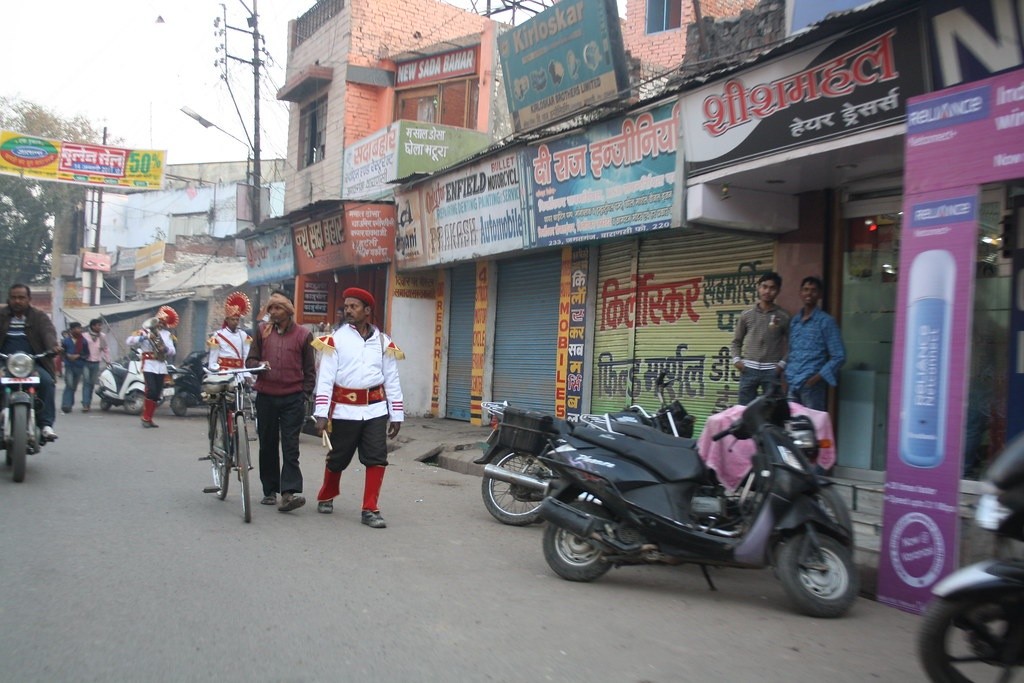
[343,289,374,312]
[157,304,180,329]
[226,290,250,321]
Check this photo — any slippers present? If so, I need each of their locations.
[279,495,305,512]
[261,491,277,506]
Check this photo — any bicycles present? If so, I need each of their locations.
[194,360,271,524]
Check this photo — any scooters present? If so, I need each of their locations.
[92,349,210,418]
[916,431,1023,683]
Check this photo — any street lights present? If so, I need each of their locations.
[178,104,264,331]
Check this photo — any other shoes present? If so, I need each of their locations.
[40,425,57,443]
[141,418,158,429]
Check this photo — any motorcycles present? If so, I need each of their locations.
[471,350,859,619]
[0,347,65,483]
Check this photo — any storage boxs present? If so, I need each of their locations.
[499,406,554,451]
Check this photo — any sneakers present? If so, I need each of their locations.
[318,497,334,513]
[361,508,385,527]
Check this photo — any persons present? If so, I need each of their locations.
[311,287,405,528]
[784,276,846,478]
[81,319,111,412]
[731,271,790,406]
[208,309,253,438]
[0,283,64,441]
[126,321,177,427]
[246,291,317,512]
[60,322,90,413]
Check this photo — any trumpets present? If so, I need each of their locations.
[142,318,167,360]
